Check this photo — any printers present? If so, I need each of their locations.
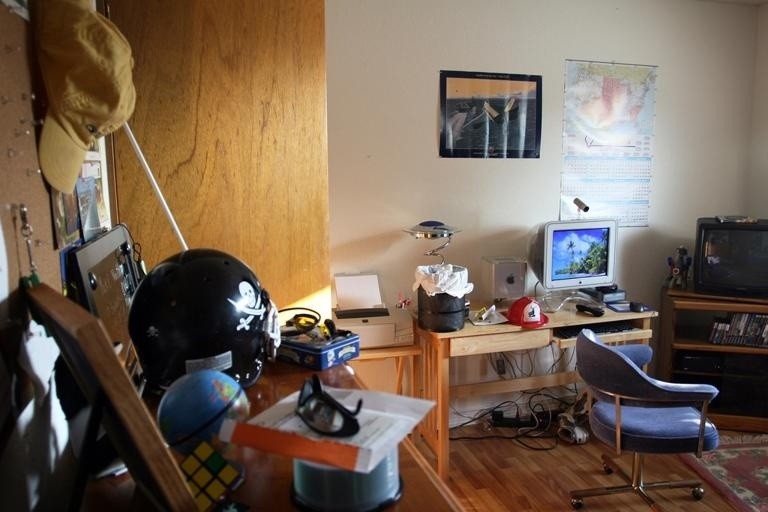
[331,270,414,350]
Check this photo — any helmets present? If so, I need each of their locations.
[126,248,283,393]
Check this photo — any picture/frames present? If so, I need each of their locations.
[63,224,137,378]
[24,277,201,512]
[438,70,542,159]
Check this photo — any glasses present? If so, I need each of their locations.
[296,374,363,439]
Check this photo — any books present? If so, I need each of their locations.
[676,328,707,344]
[708,311,768,347]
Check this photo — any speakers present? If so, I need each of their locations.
[480,256,528,307]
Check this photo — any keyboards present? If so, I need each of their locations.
[557,321,634,340]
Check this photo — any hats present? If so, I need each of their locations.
[31,2,138,197]
[508,296,548,331]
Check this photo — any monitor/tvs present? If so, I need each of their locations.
[693,218,768,299]
[70,221,147,399]
[535,219,617,312]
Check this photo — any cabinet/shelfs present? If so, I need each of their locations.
[655,283,767,433]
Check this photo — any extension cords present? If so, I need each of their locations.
[493,416,548,428]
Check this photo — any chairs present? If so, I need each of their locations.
[567,328,720,511]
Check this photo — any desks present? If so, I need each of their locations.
[79,357,467,511]
[407,296,659,482]
[343,342,421,395]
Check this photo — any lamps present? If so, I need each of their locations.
[401,219,471,307]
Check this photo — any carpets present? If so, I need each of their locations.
[677,431,766,512]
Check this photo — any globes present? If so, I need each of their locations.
[157,368,251,494]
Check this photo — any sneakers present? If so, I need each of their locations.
[559,396,595,426]
[557,414,589,446]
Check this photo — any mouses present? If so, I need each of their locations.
[630,301,644,313]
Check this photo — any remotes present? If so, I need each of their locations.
[576,304,604,317]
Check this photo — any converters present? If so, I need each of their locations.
[496,359,505,374]
[491,410,503,420]
